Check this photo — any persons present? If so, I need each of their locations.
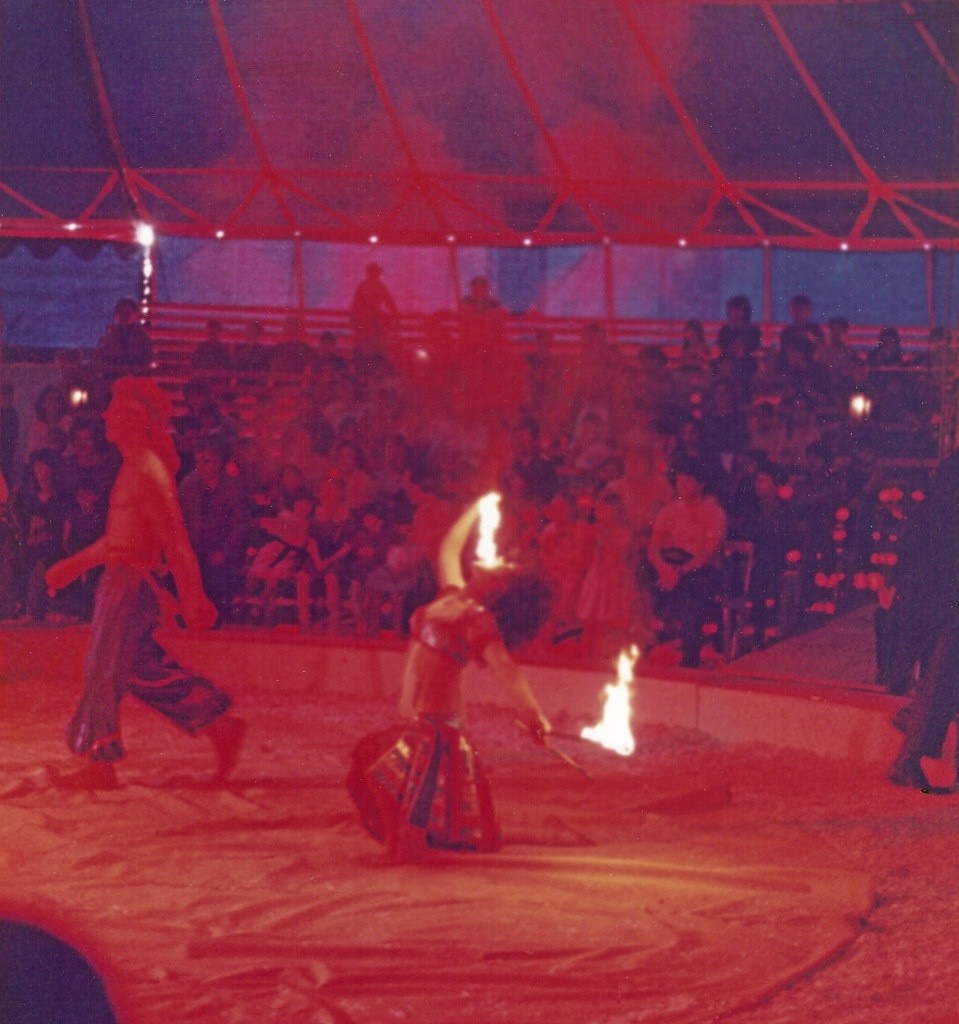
[41,371,246,790]
[883,451,959,796]
[339,489,553,867]
[0,296,959,691]
[461,279,509,337]
[349,262,400,338]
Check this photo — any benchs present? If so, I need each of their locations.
[139,297,933,667]
